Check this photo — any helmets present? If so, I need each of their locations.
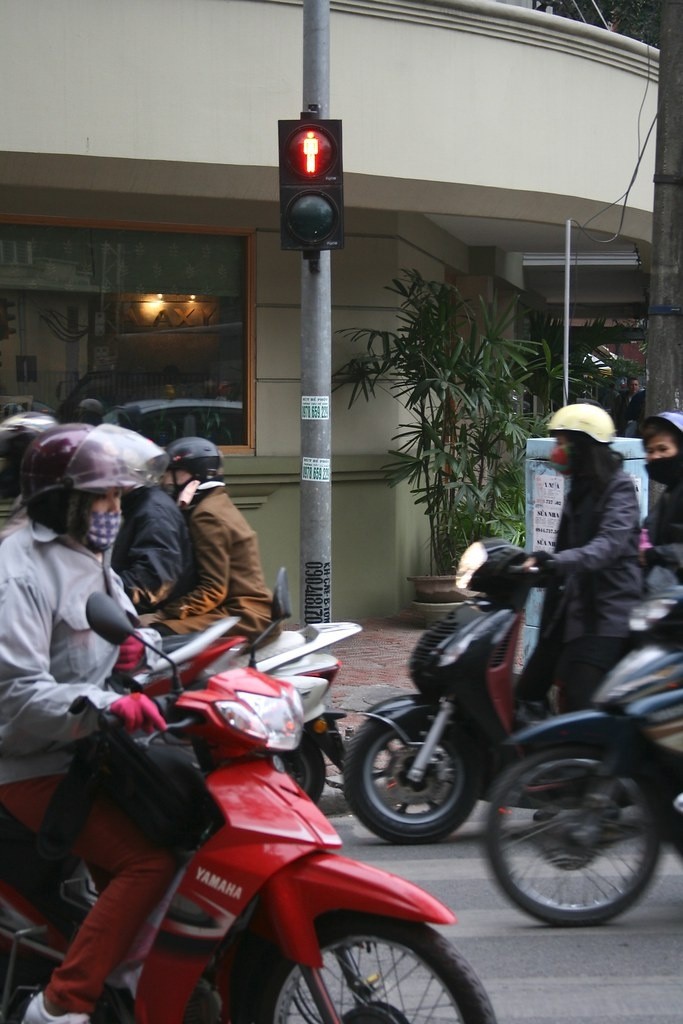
[642,412,683,441]
[0,408,56,460]
[546,404,620,445]
[162,437,226,489]
[79,398,105,417]
[17,426,120,510]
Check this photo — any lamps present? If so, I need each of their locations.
[523,251,641,265]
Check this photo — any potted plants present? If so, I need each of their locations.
[331,268,542,602]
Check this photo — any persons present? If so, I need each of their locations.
[511,379,683,845]
[0,413,280,1024]
[4,398,156,443]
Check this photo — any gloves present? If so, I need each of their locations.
[110,628,147,674]
[108,694,168,737]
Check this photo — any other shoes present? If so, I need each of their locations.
[17,989,90,1024]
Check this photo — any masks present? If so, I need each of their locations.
[77,508,123,553]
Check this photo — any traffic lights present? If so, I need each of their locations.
[280,118,346,249]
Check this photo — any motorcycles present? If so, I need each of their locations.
[340,535,683,845]
[115,614,326,809]
[0,567,498,1024]
[230,621,363,773]
[478,595,683,928]
